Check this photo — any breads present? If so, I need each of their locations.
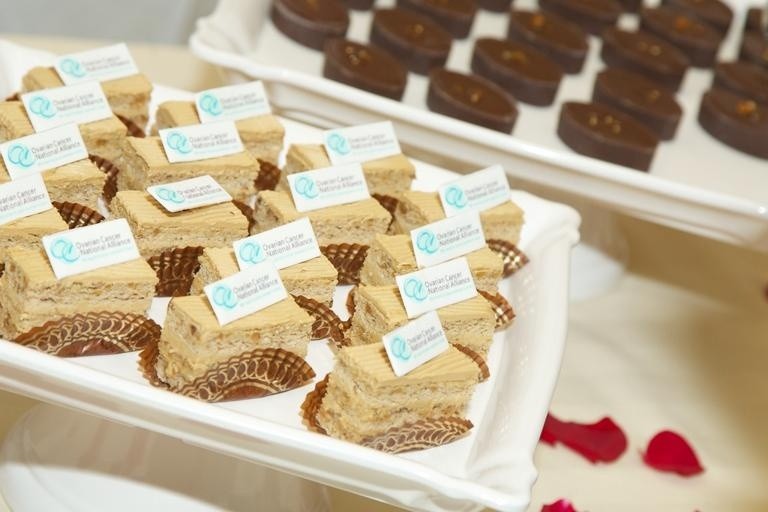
[341,283,496,361]
[0,205,68,262]
[315,340,481,446]
[0,99,128,169]
[156,293,315,386]
[20,67,153,135]
[1,245,160,341]
[107,190,251,257]
[391,189,525,248]
[1,160,107,210]
[275,143,417,196]
[186,245,340,309]
[115,136,261,196]
[251,189,393,245]
[357,233,506,284]
[147,100,287,169]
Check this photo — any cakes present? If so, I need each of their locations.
[661,1,732,41]
[476,0,516,14]
[426,68,518,136]
[508,11,588,74]
[739,38,768,67]
[698,90,767,159]
[713,62,768,107]
[538,0,624,37]
[370,7,451,77]
[600,26,689,90]
[399,1,476,41]
[270,1,350,51]
[558,102,659,172]
[638,7,719,67]
[593,68,682,140]
[349,0,374,10]
[321,34,407,102]
[471,38,563,106]
